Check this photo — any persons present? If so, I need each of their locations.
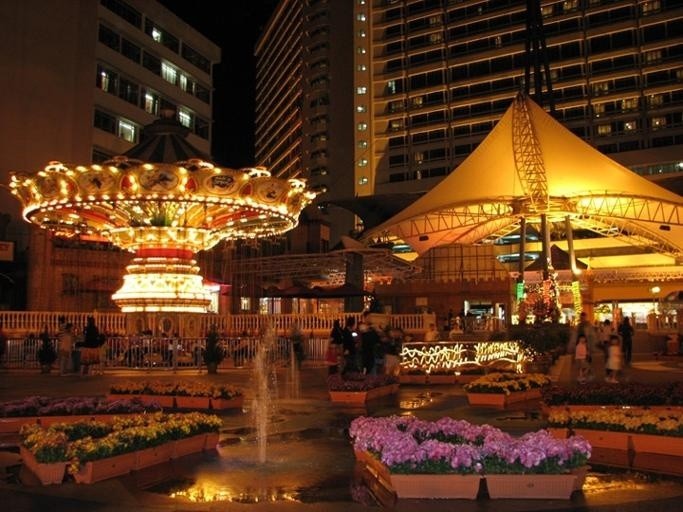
[447,309,489,328]
[57,316,100,374]
[573,313,635,384]
[425,322,438,343]
[322,311,407,380]
[205,326,225,370]
[125,325,184,368]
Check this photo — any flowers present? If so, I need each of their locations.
[348,411,594,475]
[0,372,243,484]
[326,372,401,402]
[465,366,682,450]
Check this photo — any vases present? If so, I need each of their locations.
[351,444,593,500]
